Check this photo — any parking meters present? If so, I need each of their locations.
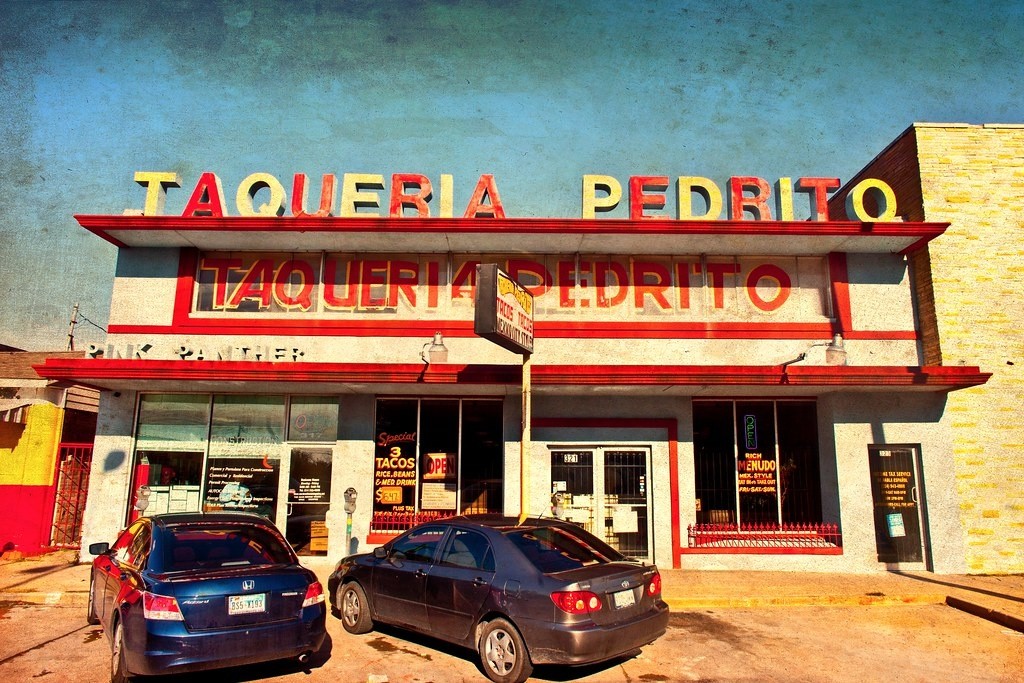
[344,487,358,555]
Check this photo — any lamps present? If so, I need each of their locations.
[418,331,447,364]
[805,333,847,366]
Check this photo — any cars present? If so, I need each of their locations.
[88,509,327,683]
[326,511,670,683]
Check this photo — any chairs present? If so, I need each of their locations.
[448,535,483,569]
[208,546,228,560]
[174,546,195,561]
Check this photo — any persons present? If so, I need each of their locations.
[219,473,253,512]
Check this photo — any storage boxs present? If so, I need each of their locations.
[706,510,736,523]
